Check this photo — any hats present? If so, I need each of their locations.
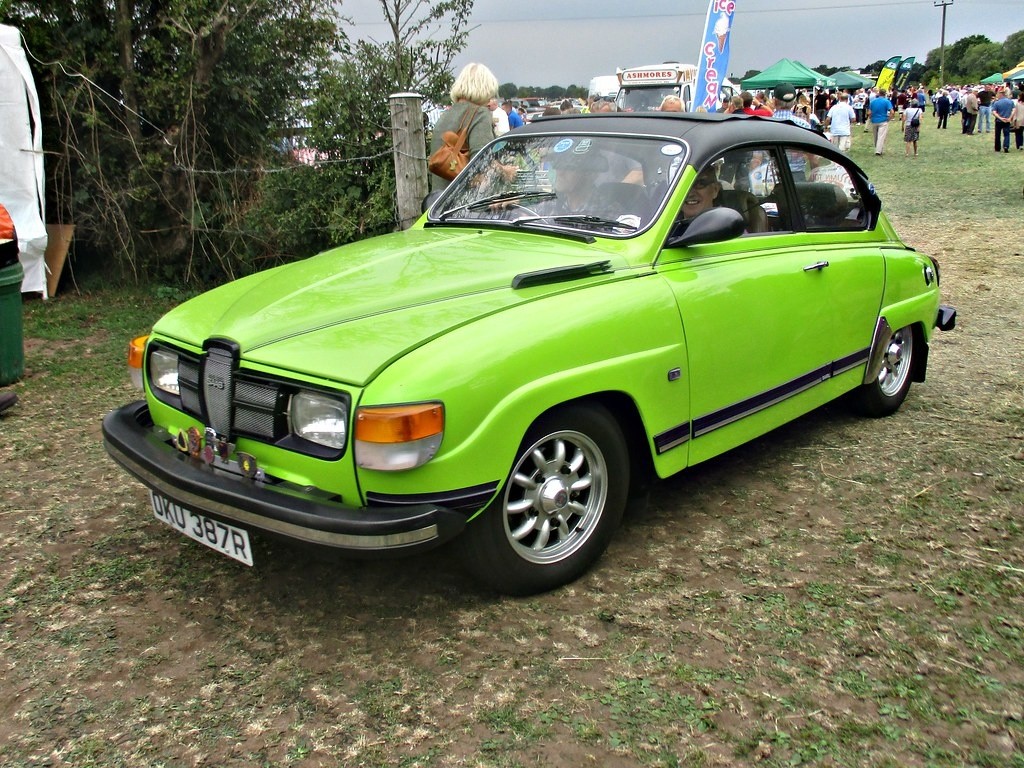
[774,82,796,103]
[909,99,918,105]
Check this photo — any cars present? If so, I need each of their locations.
[97,109,958,596]
[497,96,588,124]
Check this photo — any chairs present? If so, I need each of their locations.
[715,188,767,235]
[599,182,647,220]
[775,182,848,225]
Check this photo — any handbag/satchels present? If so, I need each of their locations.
[911,119,920,128]
[428,106,481,190]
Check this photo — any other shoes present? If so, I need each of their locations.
[1004,147,1008,152]
[906,154,908,156]
[967,132,974,135]
[1019,146,1024,151]
[914,154,919,156]
[986,130,990,133]
[978,131,981,133]
[876,153,881,156]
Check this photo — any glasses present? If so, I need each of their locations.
[692,179,718,190]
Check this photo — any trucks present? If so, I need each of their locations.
[588,74,620,101]
[613,61,741,112]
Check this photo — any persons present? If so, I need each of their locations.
[830,87,880,133]
[721,82,820,191]
[541,101,582,116]
[428,62,519,195]
[680,164,720,219]
[931,83,1024,153]
[815,89,830,123]
[589,95,615,113]
[488,100,527,138]
[889,86,925,121]
[660,95,684,112]
[901,100,924,156]
[869,89,894,155]
[825,95,855,152]
[488,151,621,224]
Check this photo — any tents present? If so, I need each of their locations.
[1002,61,1024,82]
[793,60,836,90]
[739,58,824,88]
[830,71,875,89]
[980,73,1003,84]
